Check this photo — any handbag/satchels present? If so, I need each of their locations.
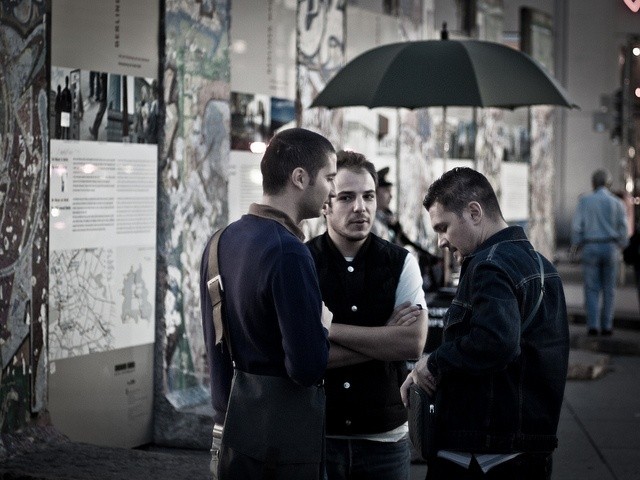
[405,251,545,465]
[207,226,230,479]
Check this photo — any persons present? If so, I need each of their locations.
[299,149,430,479]
[200,127,338,480]
[572,166,632,338]
[54,84,64,138]
[61,75,72,140]
[400,166,569,479]
[370,167,394,243]
[137,84,149,143]
[257,100,268,143]
[148,79,160,143]
[88,71,108,141]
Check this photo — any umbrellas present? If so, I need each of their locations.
[302,20,579,171]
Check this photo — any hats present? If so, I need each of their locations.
[377,166,394,188]
